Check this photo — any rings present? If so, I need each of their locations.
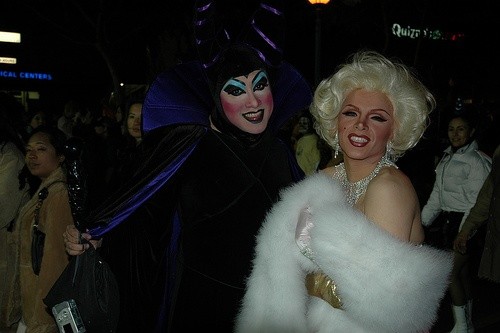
[64,243,67,247]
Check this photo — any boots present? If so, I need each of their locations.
[449,304,467,333]
[465,299,474,333]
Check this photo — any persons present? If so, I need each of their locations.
[422,116,493,333]
[448,145,500,333]
[8,128,72,333]
[62,44,312,333]
[237,52,452,333]
[0,91,143,333]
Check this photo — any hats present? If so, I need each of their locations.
[208,42,274,120]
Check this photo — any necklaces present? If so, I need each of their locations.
[332,160,398,207]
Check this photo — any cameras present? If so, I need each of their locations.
[52,299,86,333]
